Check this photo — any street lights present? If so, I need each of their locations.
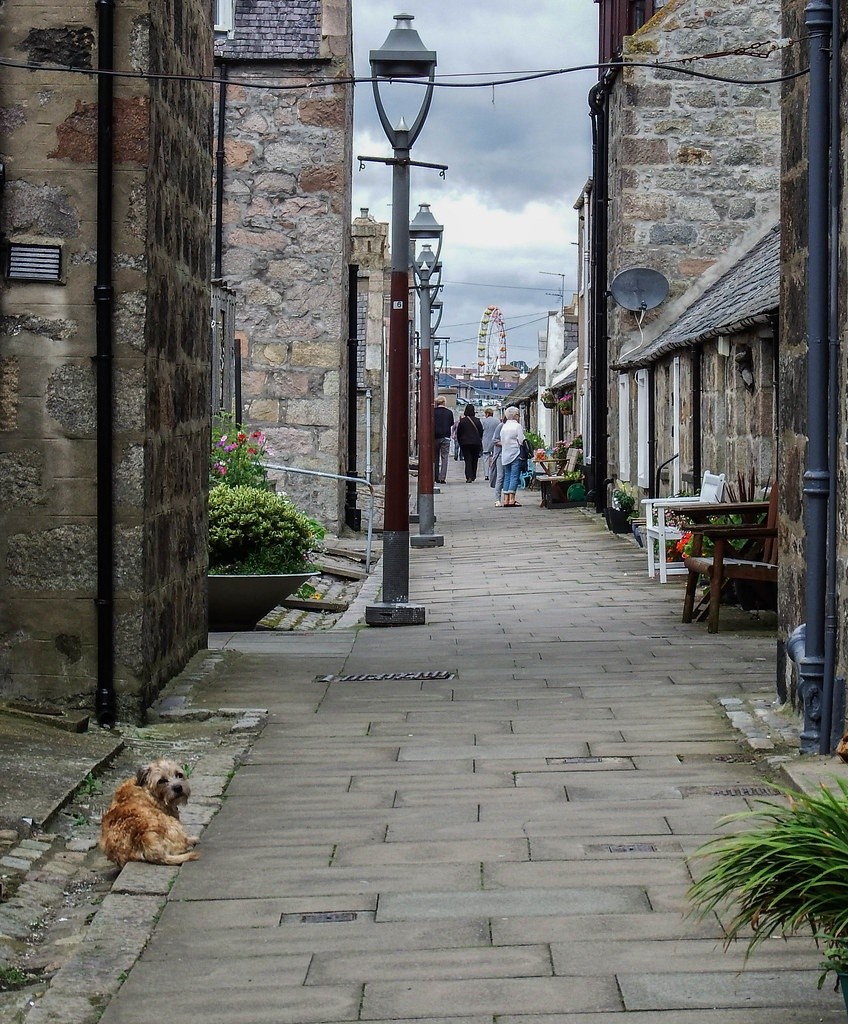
[358,13,448,628]
[406,203,448,548]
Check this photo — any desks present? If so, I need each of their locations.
[668,501,770,623]
[532,458,570,507]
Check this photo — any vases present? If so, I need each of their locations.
[560,407,572,415]
[540,390,556,403]
[543,402,557,409]
[631,516,647,547]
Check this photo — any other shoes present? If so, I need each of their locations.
[495,501,503,507]
[436,480,446,484]
[466,479,472,483]
[454,457,458,461]
[484,476,489,480]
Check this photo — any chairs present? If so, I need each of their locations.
[566,447,580,473]
[640,470,724,583]
[671,481,778,634]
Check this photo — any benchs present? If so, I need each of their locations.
[536,474,585,507]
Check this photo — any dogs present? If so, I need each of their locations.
[98,757,202,864]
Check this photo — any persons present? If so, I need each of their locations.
[433,396,454,483]
[452,404,527,507]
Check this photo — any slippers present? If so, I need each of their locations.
[504,501,522,507]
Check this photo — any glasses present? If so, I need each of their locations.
[516,413,520,417]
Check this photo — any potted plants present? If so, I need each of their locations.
[206,483,323,632]
[604,488,638,534]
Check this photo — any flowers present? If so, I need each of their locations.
[551,440,573,453]
[558,395,572,407]
[215,428,269,489]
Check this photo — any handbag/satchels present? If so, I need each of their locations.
[479,441,483,458]
